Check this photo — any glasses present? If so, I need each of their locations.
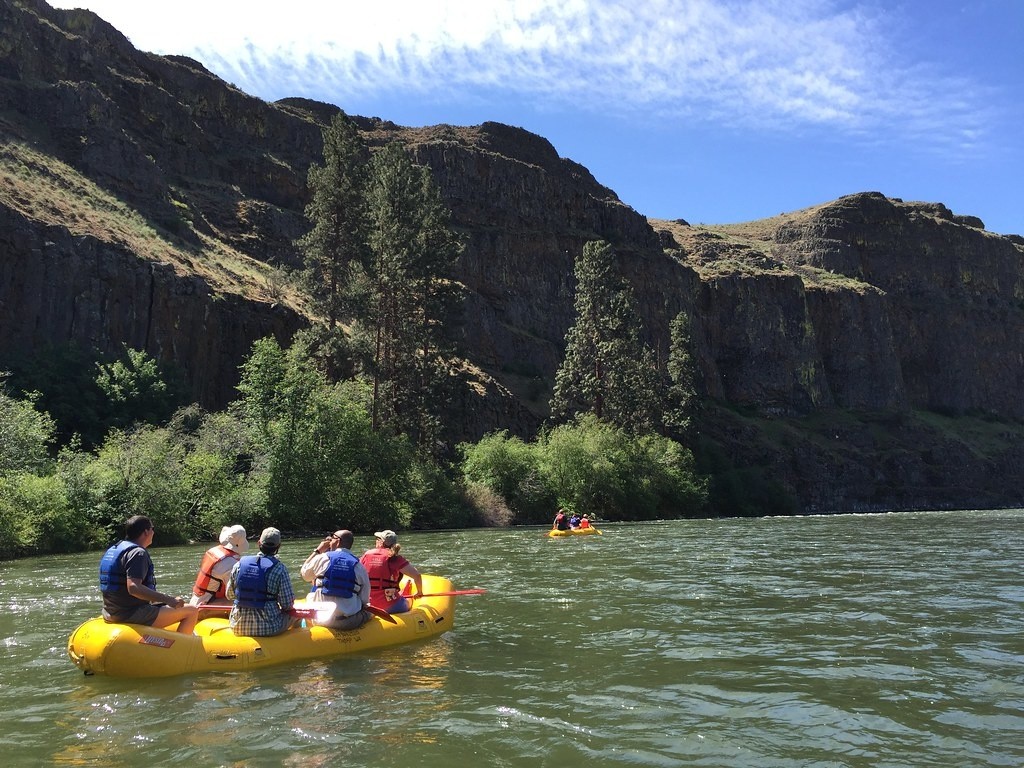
[329,532,342,541]
[150,526,155,531]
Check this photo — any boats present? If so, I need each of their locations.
[543,521,603,536]
[68,574,455,678]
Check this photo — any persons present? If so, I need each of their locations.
[191,525,249,619]
[581,514,590,528]
[553,510,570,530]
[570,514,581,530]
[226,527,301,637]
[99,516,198,633]
[359,530,423,614]
[301,530,374,631]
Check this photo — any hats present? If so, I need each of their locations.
[259,526,281,548]
[219,525,249,554]
[374,530,397,546]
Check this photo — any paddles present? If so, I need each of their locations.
[402,589,486,600]
[197,605,318,618]
[365,604,398,626]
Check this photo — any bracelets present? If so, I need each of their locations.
[313,549,320,554]
